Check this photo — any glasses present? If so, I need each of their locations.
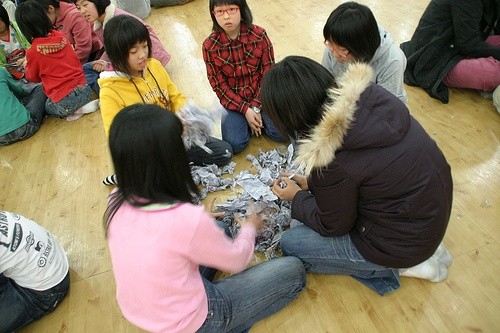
[323,38,353,59]
[211,6,239,16]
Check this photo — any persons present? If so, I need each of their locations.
[399,1,500,115]
[0,209,71,333]
[100,103,306,333]
[260,55,453,296]
[97,14,233,186]
[38,0,106,66]
[15,1,101,122]
[0,63,48,147]
[202,0,290,154]
[0,0,31,81]
[74,0,171,98]
[319,0,408,106]
